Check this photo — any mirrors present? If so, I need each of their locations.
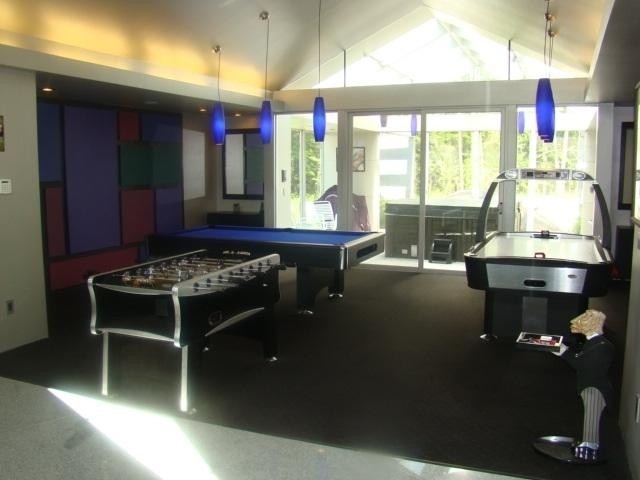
[222,128,264,200]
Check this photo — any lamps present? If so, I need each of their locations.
[380,63,387,127]
[518,0,558,143]
[209,45,226,146]
[259,11,273,144]
[313,0,326,142]
[411,80,417,136]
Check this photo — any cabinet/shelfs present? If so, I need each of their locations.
[207,210,264,227]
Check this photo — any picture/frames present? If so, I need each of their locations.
[618,121,635,210]
[336,147,365,172]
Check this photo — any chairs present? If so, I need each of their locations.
[300,200,337,231]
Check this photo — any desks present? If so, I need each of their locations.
[144,224,386,315]
